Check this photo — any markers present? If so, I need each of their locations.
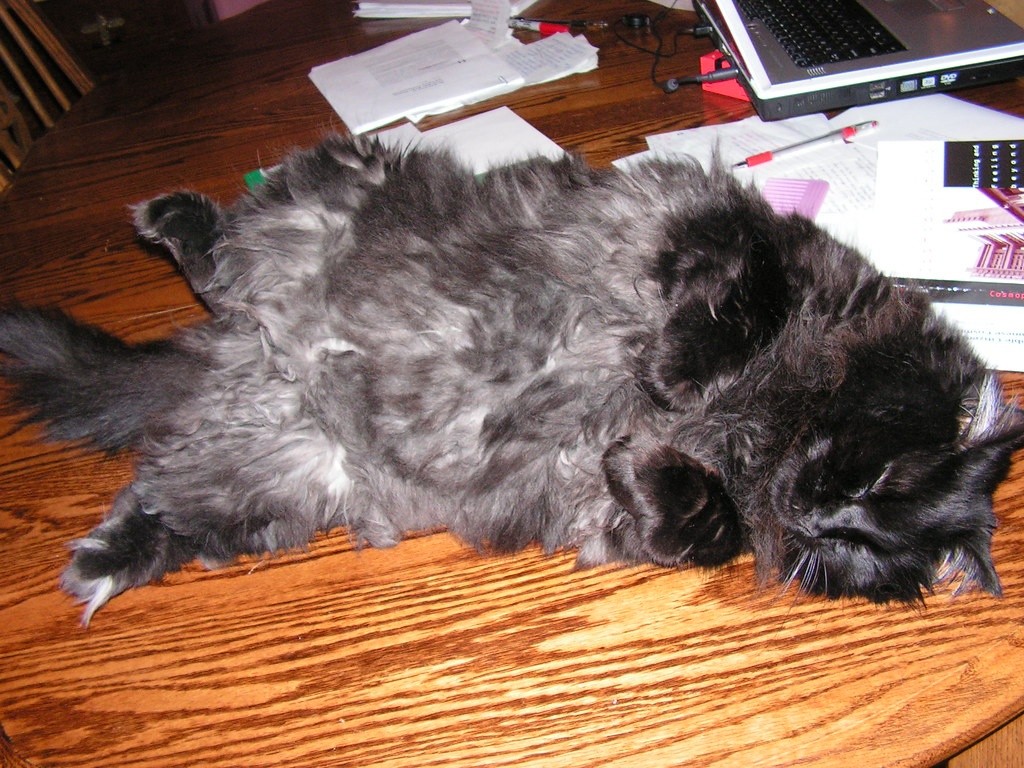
[510,18,569,36]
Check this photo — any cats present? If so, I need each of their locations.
[0,117,1024,631]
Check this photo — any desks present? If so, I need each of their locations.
[0,0,1023,768]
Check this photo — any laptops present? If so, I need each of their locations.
[693,0,1024,122]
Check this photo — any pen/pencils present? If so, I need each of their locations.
[730,121,882,173]
[518,16,610,27]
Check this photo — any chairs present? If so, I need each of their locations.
[0,1,89,190]
